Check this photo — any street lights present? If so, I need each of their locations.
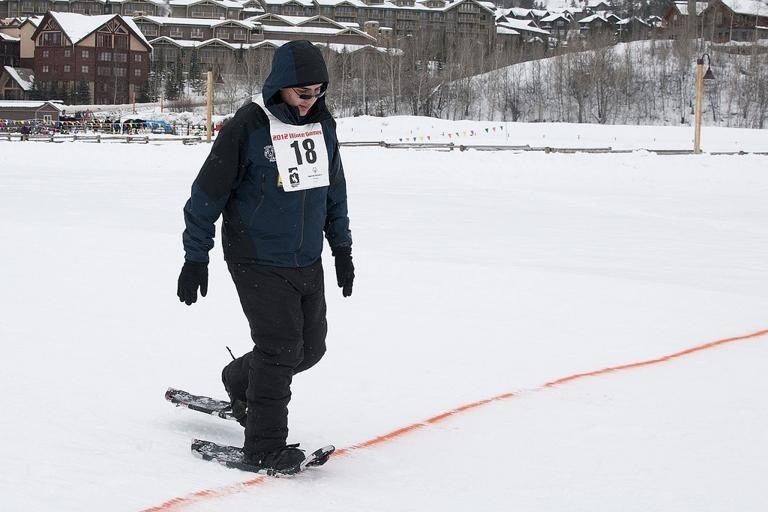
[395,32,412,73]
[693,53,717,153]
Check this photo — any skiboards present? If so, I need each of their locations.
[165,388,335,476]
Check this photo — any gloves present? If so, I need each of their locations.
[335,253,354,297]
[177,260,208,305]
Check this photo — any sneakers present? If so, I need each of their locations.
[243,442,304,469]
[222,360,247,415]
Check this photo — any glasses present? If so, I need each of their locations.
[292,87,325,99]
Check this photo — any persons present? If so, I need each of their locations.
[176,38,356,473]
[0,108,232,142]
